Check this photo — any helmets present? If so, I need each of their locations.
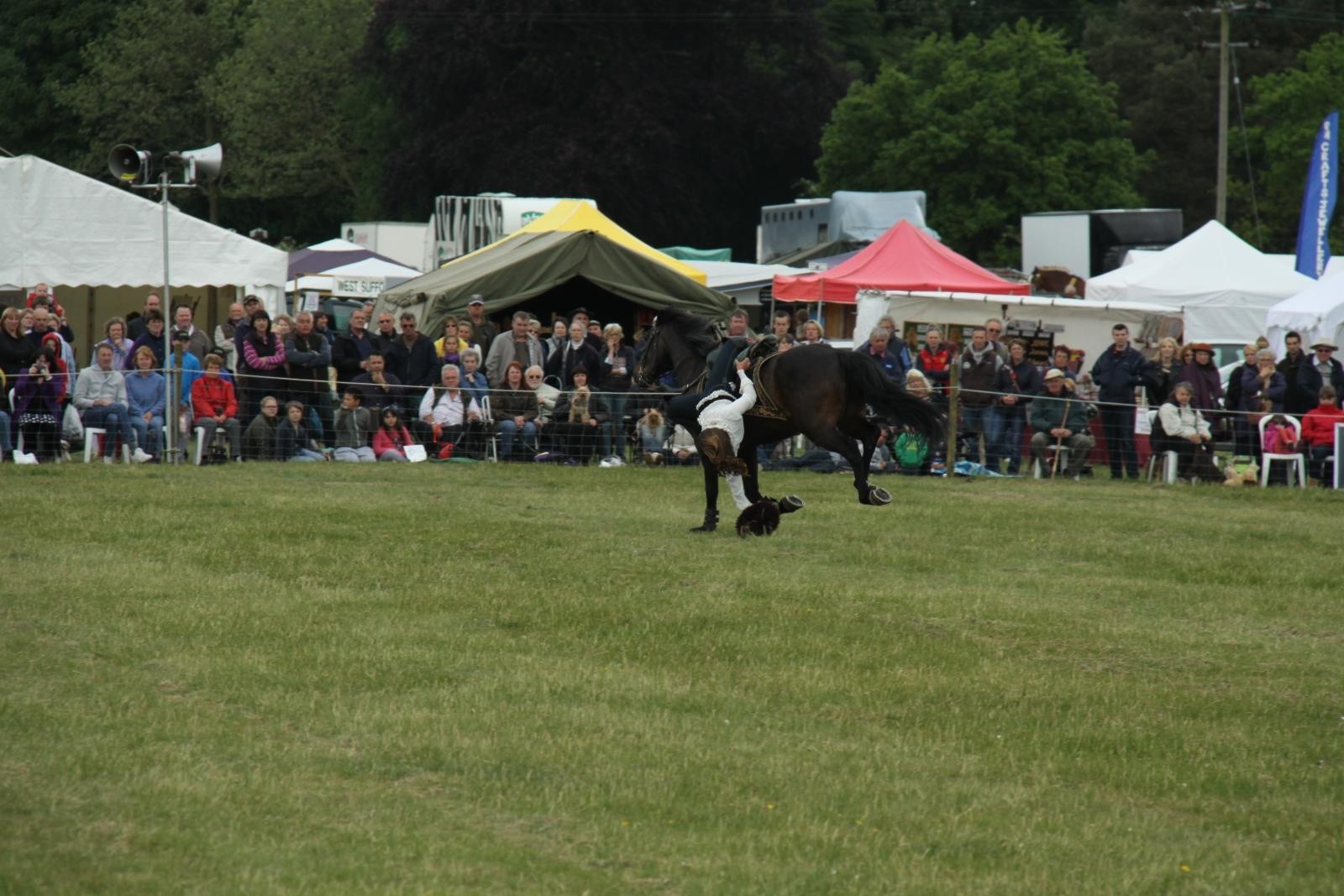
[737,503,779,539]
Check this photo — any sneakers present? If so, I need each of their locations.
[131,446,154,464]
[103,456,113,466]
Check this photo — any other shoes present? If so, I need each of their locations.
[1287,439,1303,452]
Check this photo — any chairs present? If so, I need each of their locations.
[482,394,539,463]
[1146,410,1179,486]
[83,427,138,468]
[161,426,171,463]
[9,388,71,461]
[189,391,243,464]
[1025,403,1081,483]
[1257,413,1307,488]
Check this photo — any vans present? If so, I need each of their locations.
[287,294,364,333]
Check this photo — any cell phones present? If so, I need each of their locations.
[34,365,45,371]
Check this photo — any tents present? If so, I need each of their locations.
[0,152,1344,367]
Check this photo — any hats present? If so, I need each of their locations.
[174,330,189,343]
[470,293,484,307]
[210,347,226,358]
[587,321,600,327]
[569,307,594,320]
[1309,338,1339,351]
[1192,344,1217,357]
[243,295,258,307]
[1045,368,1065,381]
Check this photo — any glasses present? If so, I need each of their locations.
[528,375,544,379]
[378,320,394,324]
[444,324,457,328]
[987,328,1003,334]
[1317,349,1333,353]
[402,322,417,328]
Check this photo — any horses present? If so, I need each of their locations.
[638,309,946,533]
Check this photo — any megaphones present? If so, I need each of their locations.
[108,144,151,181]
[180,142,223,181]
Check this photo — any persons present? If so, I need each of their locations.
[663,334,758,512]
[0,283,1344,491]
